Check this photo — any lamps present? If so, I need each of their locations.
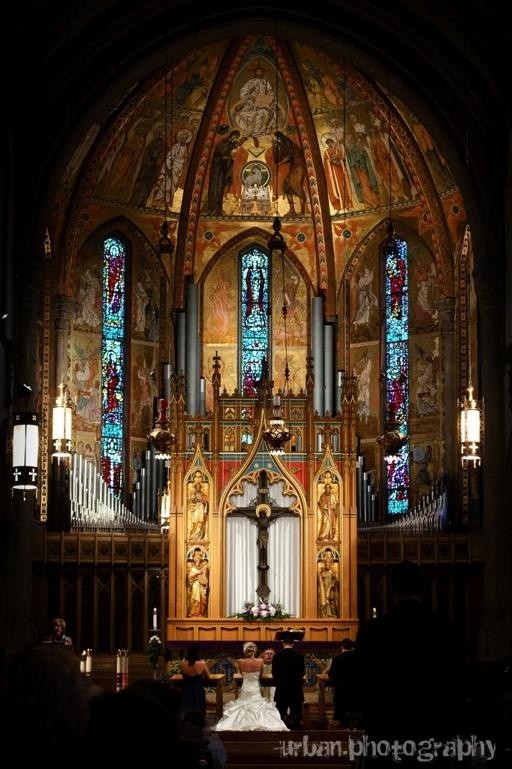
[9,412,40,517]
[456,230,486,470]
[51,223,73,467]
[147,41,179,460]
[375,50,410,465]
[261,35,293,457]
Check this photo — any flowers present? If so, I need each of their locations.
[227,597,296,622]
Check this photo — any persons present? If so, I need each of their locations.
[258,649,277,697]
[318,551,340,619]
[350,557,481,768]
[272,634,305,729]
[328,638,357,729]
[95,59,413,218]
[1,656,229,768]
[43,618,73,645]
[214,641,291,733]
[317,483,339,540]
[190,482,208,539]
[185,547,209,617]
[179,647,213,723]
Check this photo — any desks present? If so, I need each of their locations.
[170,674,224,719]
[233,673,306,725]
[315,674,335,725]
